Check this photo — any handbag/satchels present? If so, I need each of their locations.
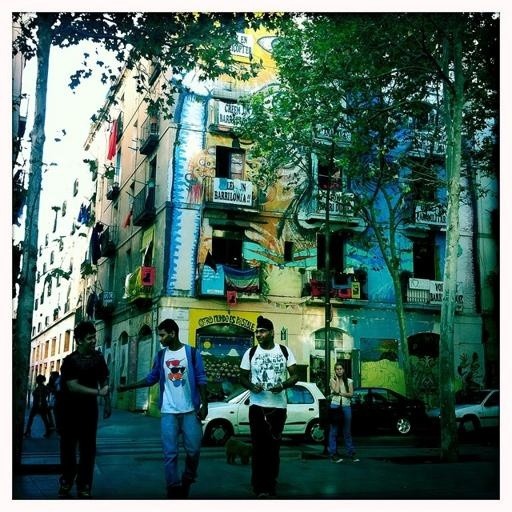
[328,407,344,417]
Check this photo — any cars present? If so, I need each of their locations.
[325,387,425,435]
[428,388,500,434]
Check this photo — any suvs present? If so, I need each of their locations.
[199,382,324,445]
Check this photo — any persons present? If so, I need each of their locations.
[23,365,64,438]
[115,318,209,500]
[327,361,361,465]
[239,315,299,500]
[57,320,111,500]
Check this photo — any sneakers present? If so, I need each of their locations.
[77,490,93,499]
[332,454,343,463]
[58,482,73,497]
[351,454,360,463]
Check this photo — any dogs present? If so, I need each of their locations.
[224,436,253,465]
